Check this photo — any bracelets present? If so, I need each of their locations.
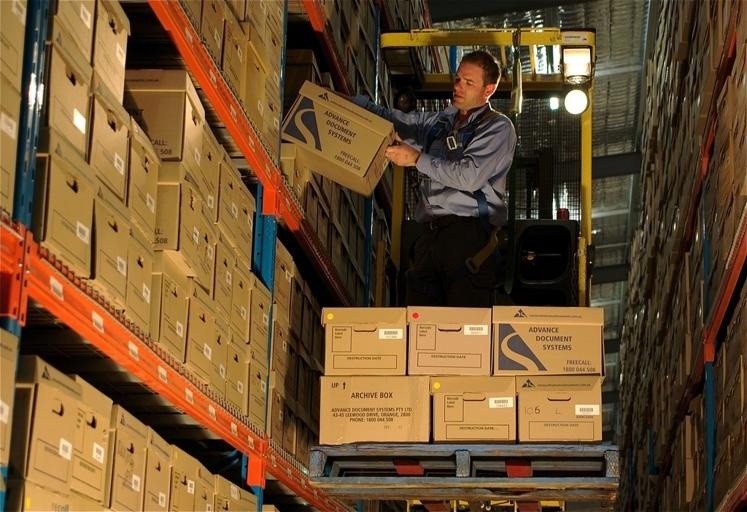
[414,153,420,164]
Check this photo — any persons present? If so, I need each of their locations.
[318,49,518,307]
